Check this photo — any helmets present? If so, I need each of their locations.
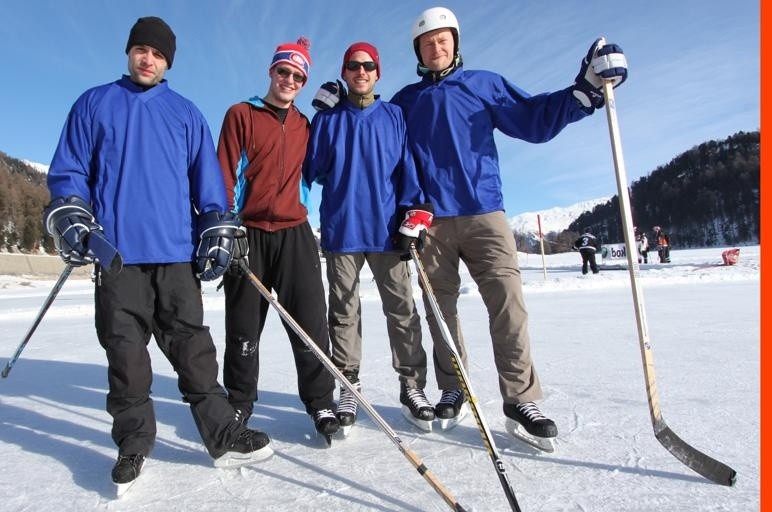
[414,7,460,66]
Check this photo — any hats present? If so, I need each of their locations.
[126,16,177,68]
[342,42,380,81]
[269,38,311,86]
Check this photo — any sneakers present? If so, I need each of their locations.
[503,401,558,438]
[401,386,436,420]
[436,389,468,418]
[314,409,339,434]
[112,452,145,483]
[227,399,255,424]
[229,430,270,454]
[336,374,361,425]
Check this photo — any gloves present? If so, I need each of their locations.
[195,210,240,282]
[227,225,250,277]
[43,196,103,267]
[392,204,435,260]
[572,36,628,109]
[313,80,348,110]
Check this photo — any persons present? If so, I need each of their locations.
[218,37,348,449]
[642,234,649,264]
[313,6,628,452]
[654,225,668,263]
[43,16,276,498]
[301,41,438,435]
[571,228,604,281]
[633,226,644,265]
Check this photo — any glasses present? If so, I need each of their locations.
[274,66,306,82]
[345,61,377,71]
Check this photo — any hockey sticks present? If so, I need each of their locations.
[595,36,741,486]
[1,231,124,379]
